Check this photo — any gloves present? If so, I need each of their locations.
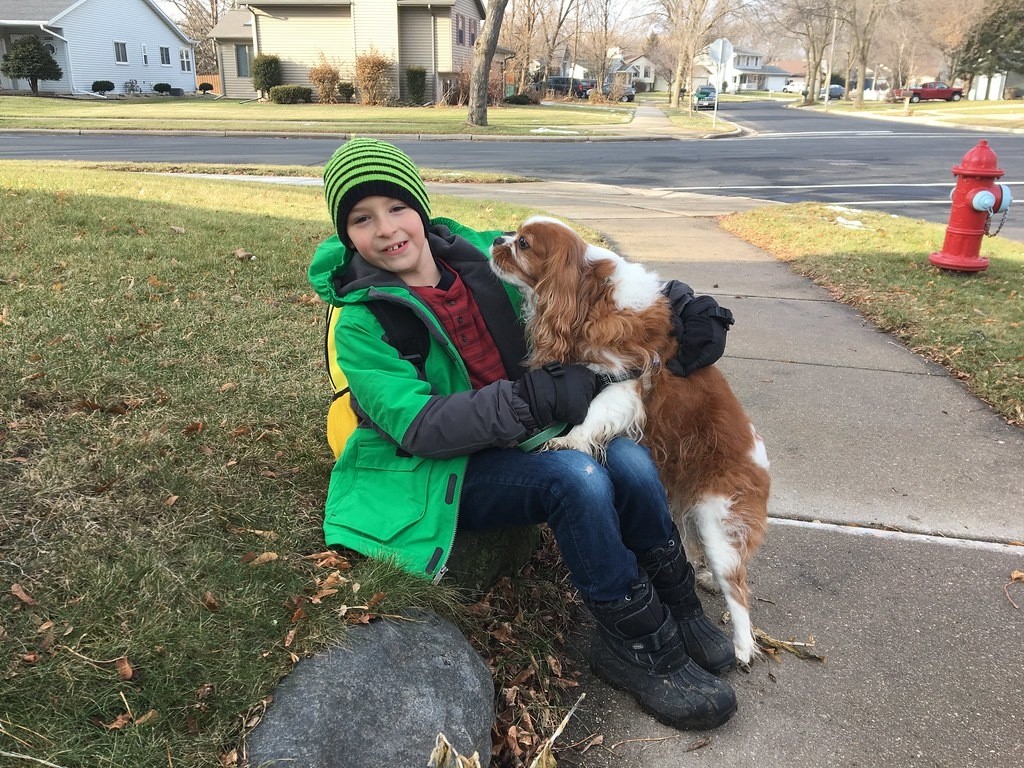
[518,360,601,429]
[666,295,735,379]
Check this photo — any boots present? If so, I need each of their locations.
[628,522,736,673]
[582,564,738,731]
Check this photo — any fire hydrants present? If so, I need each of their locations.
[929,140,1013,272]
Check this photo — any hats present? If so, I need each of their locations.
[323,139,432,247]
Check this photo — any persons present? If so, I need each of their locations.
[306,139,736,728]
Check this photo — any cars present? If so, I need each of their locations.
[802,85,845,100]
[527,76,634,103]
[693,84,718,110]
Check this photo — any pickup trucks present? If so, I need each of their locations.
[894,82,964,103]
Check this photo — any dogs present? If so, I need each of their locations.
[489,214,772,668]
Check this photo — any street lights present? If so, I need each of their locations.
[806,6,838,108]
